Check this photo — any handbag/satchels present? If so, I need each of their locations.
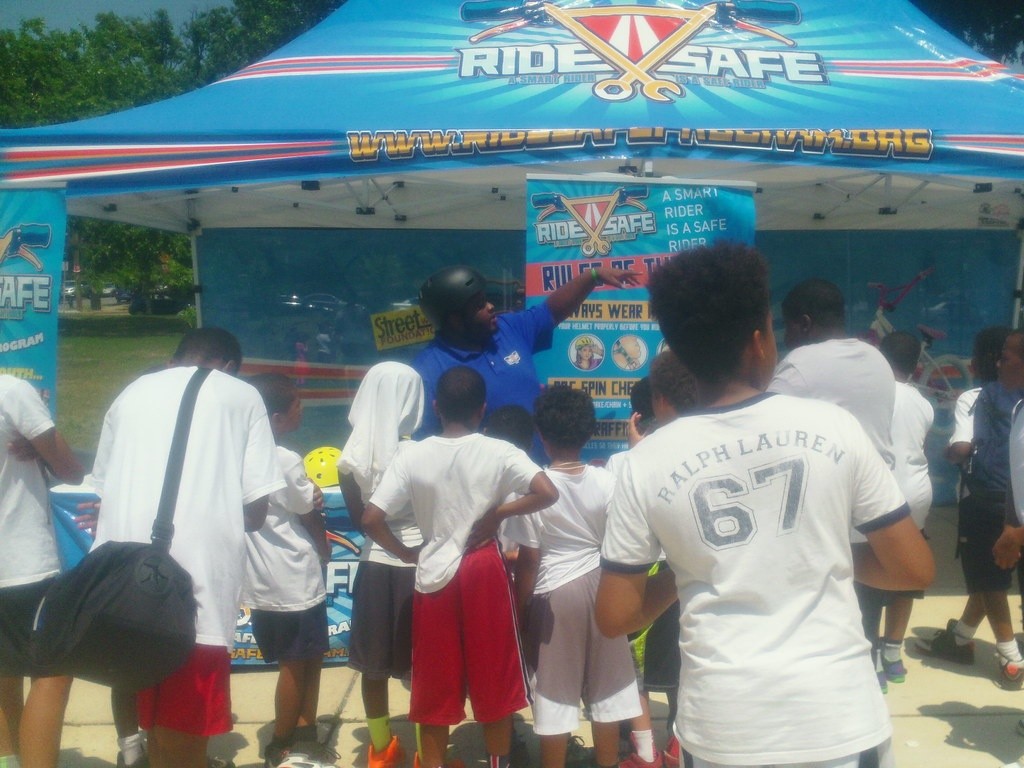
[66,542,198,691]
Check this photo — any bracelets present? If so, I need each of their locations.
[589,267,603,287]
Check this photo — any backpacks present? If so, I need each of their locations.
[955,381,1017,501]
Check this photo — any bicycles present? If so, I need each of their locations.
[858,268,975,436]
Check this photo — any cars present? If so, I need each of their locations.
[60,280,85,298]
[114,286,135,301]
[101,281,116,296]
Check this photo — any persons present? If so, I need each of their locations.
[914,323,1024,736]
[358,364,559,768]
[412,263,643,475]
[591,239,933,768]
[337,360,426,768]
[75,327,287,768]
[765,280,932,693]
[244,372,335,768]
[512,385,644,768]
[619,350,696,446]
[1,372,86,768]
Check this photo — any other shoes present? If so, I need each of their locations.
[117,750,149,768]
[878,667,888,693]
[883,659,908,682]
[208,756,236,768]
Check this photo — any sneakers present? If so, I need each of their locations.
[502,736,531,768]
[566,736,596,766]
[613,753,662,768]
[994,639,1024,689]
[915,619,975,665]
[414,752,465,768]
[267,737,345,768]
[662,749,679,767]
[366,734,407,768]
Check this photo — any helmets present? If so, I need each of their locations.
[418,267,488,329]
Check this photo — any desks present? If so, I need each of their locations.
[50,473,365,672]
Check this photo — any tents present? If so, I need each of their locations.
[0,0,1023,331]
